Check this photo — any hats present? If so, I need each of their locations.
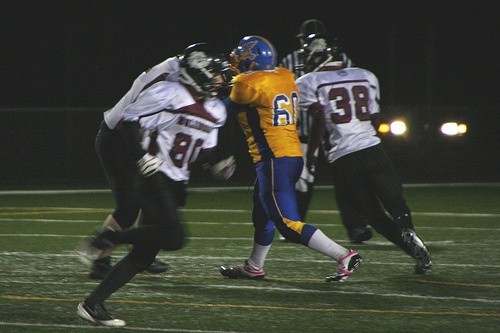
[295,19,328,38]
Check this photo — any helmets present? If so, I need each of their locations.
[177,42,235,101]
[230,36,278,72]
[296,32,339,74]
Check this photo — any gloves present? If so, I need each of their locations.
[139,151,165,177]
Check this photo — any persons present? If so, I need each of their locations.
[76,18,237,328]
[277,21,433,275]
[220,35,363,282]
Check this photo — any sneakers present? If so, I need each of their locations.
[83,227,117,259]
[413,256,434,274]
[77,299,126,327]
[90,257,114,281]
[150,259,171,272]
[348,226,374,242]
[326,249,362,282]
[219,260,265,280]
[401,229,429,258]
